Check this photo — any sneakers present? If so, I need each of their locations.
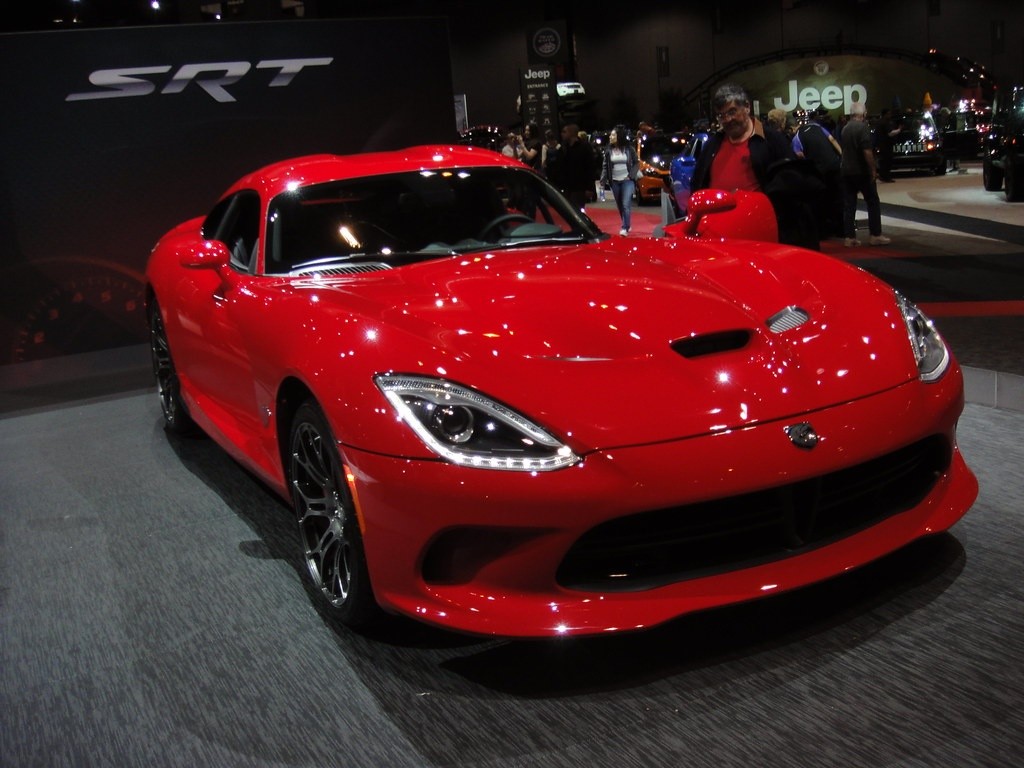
[870,234,891,245]
[844,237,861,247]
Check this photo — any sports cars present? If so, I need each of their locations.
[141,144,981,645]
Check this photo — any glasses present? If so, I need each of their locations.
[715,104,747,122]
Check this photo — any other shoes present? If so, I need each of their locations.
[949,166,961,172]
[619,227,628,236]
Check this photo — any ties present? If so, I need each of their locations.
[512,145,518,159]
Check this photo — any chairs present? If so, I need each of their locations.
[397,184,502,238]
[270,199,358,260]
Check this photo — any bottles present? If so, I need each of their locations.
[600,186,607,202]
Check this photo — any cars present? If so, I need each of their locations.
[661,129,722,221]
[635,121,697,207]
[874,97,1024,204]
[459,125,505,153]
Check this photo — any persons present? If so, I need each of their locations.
[760,102,962,245]
[501,116,719,235]
[691,85,820,250]
